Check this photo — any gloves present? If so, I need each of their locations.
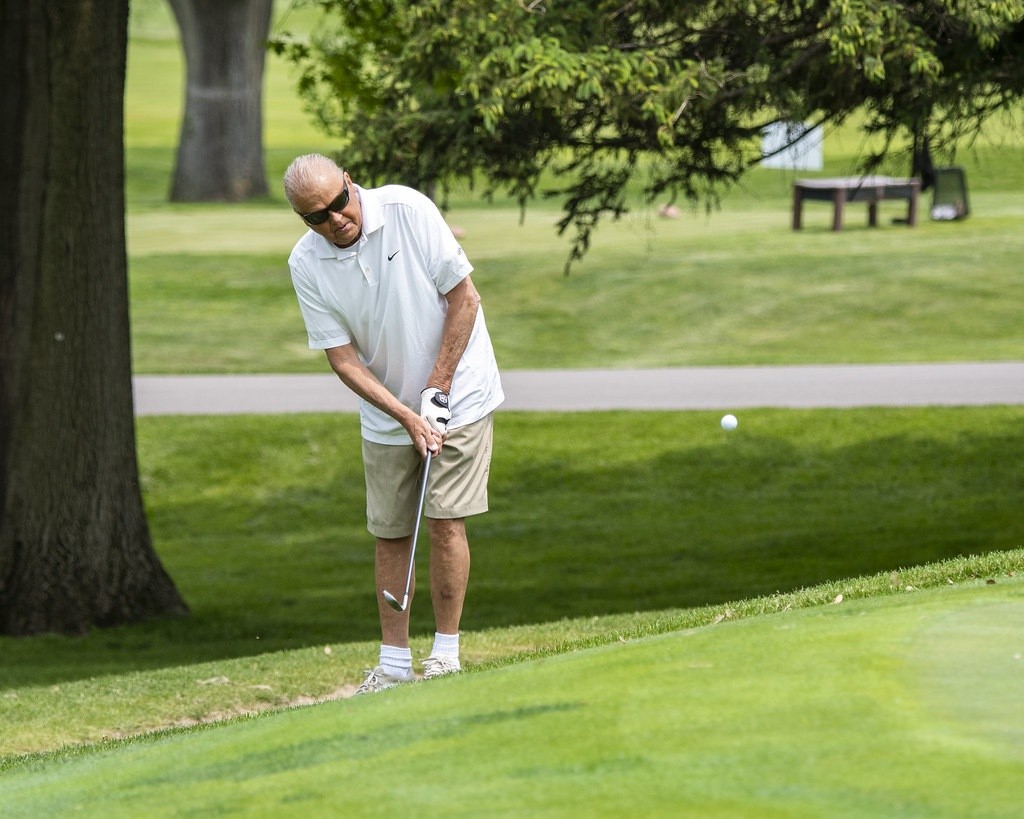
[420,387,451,437]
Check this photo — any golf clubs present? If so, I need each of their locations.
[382,448,432,613]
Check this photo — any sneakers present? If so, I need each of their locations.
[355,668,417,696]
[420,655,461,680]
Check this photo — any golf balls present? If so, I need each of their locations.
[720,414,738,432]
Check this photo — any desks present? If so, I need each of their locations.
[793,175,920,229]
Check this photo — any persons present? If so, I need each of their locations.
[283,153,505,692]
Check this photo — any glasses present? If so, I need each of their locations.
[298,167,350,225]
